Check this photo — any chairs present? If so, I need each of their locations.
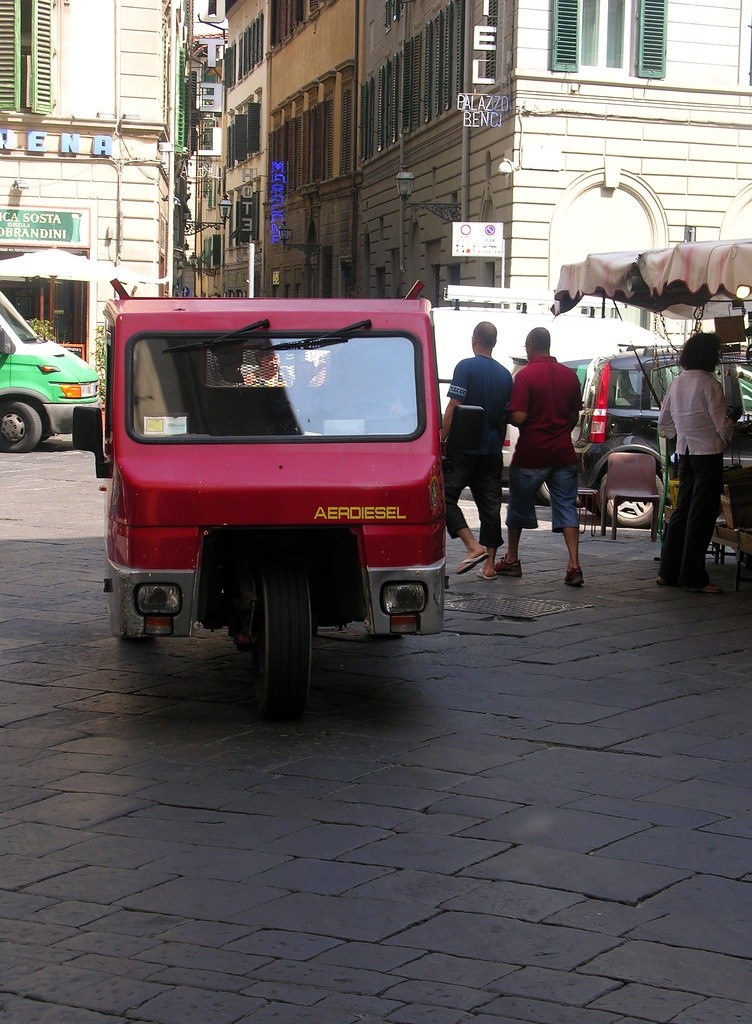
[601,452,660,543]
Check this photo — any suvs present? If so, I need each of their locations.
[556,343,752,529]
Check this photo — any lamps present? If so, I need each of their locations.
[395,163,462,211]
[277,220,320,250]
[188,252,220,273]
[184,195,233,230]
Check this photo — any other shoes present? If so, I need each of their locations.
[656,576,678,585]
[685,583,722,593]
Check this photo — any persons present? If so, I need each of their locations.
[494,327,584,585]
[656,333,733,594]
[246,343,326,386]
[440,322,513,580]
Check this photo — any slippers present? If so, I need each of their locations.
[477,568,498,580]
[455,553,489,574]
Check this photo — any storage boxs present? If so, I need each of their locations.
[665,464,752,551]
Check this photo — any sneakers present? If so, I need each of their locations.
[494,552,522,576]
[565,566,584,585]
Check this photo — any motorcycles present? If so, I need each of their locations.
[72,275,451,730]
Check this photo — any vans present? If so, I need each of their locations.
[0,291,101,454]
[430,283,680,511]
